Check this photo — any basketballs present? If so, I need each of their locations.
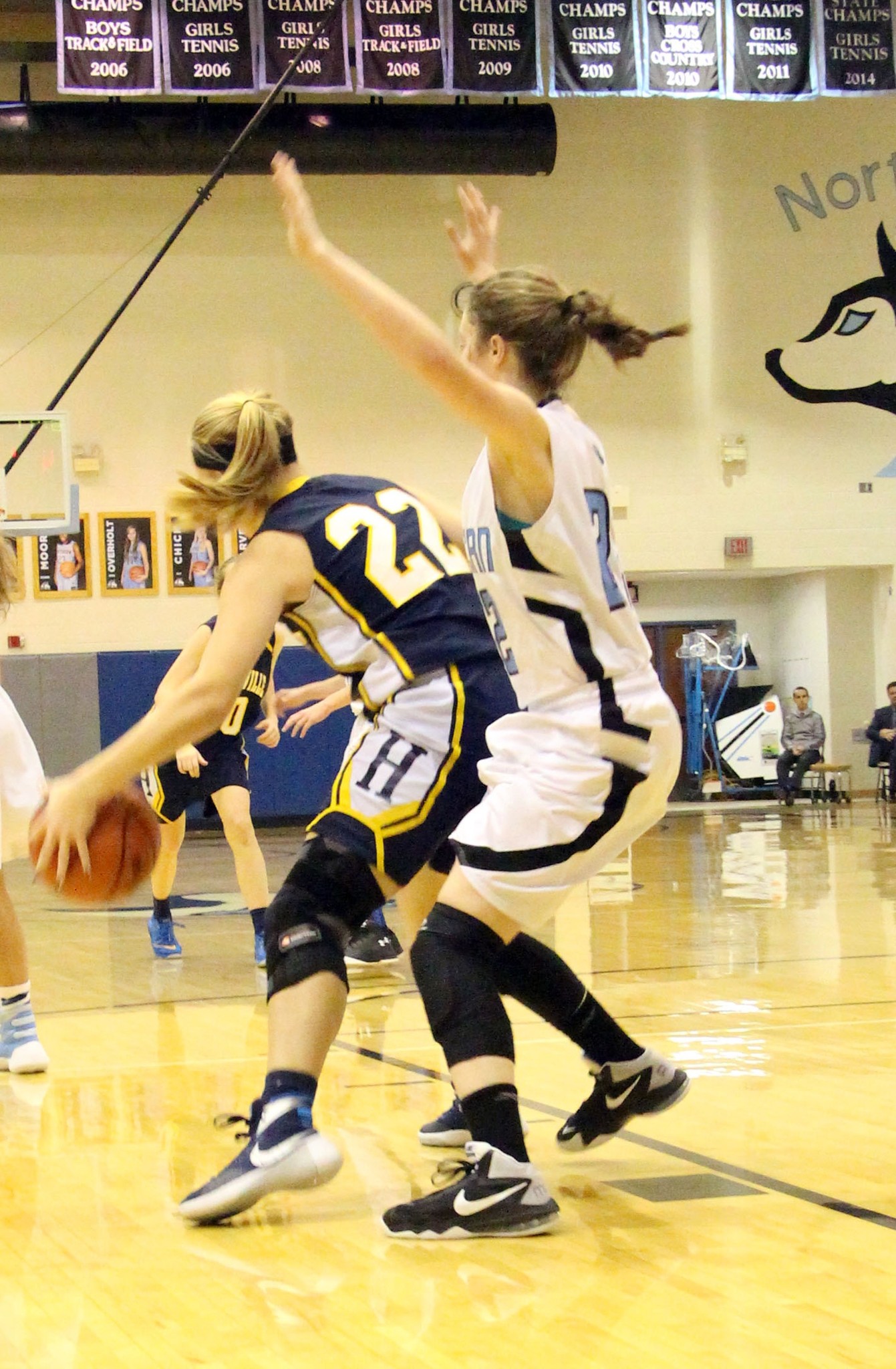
[26,782,162,902]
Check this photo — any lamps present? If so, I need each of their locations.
[72,445,103,477]
[717,437,749,464]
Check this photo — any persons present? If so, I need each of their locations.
[120,522,150,588]
[270,151,693,1241]
[27,386,529,1218]
[276,671,406,969]
[53,534,84,591]
[777,687,826,806]
[188,526,215,587]
[864,681,896,803]
[0,528,48,1075]
[141,554,284,967]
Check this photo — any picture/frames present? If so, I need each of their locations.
[30,513,95,600]
[96,512,161,597]
[164,509,227,597]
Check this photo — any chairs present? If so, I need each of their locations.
[778,744,824,805]
[875,762,891,803]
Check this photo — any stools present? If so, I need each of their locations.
[809,763,853,807]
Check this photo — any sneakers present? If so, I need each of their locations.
[149,960,183,996]
[557,1045,688,1151]
[0,1011,47,1073]
[341,925,405,964]
[254,933,265,967]
[180,1092,346,1222]
[147,915,182,959]
[381,1139,561,1240]
[418,1090,529,1144]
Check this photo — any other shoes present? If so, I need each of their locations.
[785,797,793,806]
